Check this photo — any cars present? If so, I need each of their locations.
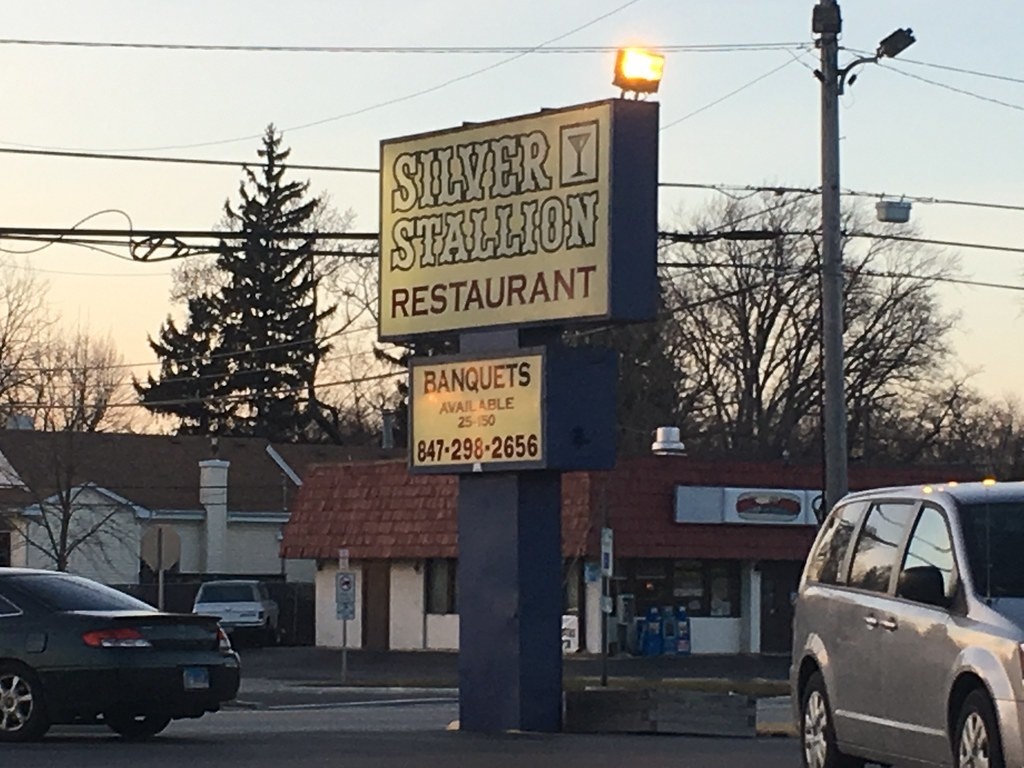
[0,566,243,744]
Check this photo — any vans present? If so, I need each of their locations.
[791,480,1024,766]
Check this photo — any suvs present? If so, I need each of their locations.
[192,578,281,646]
